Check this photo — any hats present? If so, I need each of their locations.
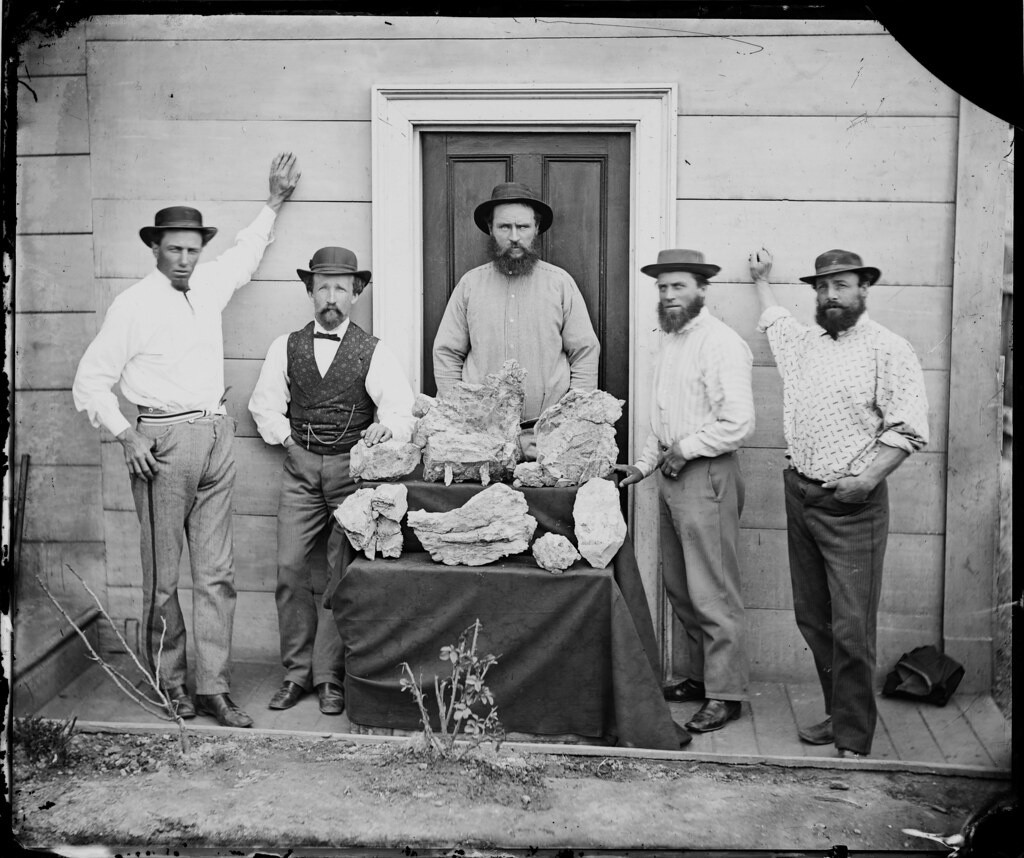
[139,206,218,248]
[799,250,881,287]
[474,181,554,236]
[297,247,372,294]
[641,249,721,280]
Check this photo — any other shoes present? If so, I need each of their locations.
[798,716,836,744]
[836,747,867,760]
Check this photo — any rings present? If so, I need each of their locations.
[671,472,677,478]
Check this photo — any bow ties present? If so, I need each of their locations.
[313,331,341,342]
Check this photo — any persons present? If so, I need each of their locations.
[613,249,755,733]
[245,246,415,717]
[72,150,303,728]
[432,182,601,433]
[748,243,931,759]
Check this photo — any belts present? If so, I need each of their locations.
[792,468,824,486]
[658,439,671,451]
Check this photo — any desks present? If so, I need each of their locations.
[322,461,693,750]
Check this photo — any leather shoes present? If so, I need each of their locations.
[161,683,196,720]
[316,681,345,714]
[684,699,741,731]
[662,679,706,701]
[269,679,310,709]
[193,692,254,727]
